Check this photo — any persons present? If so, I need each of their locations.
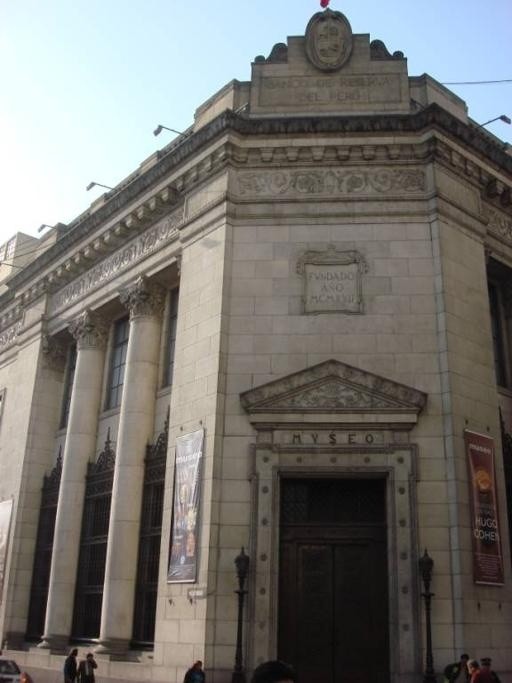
[444,653,472,682]
[480,656,501,682]
[17,673,35,683]
[465,659,490,682]
[183,660,206,683]
[76,652,98,683]
[61,648,75,682]
[249,659,301,683]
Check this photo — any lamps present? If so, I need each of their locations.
[151,120,192,145]
[85,180,120,198]
[475,113,512,135]
[37,222,67,237]
